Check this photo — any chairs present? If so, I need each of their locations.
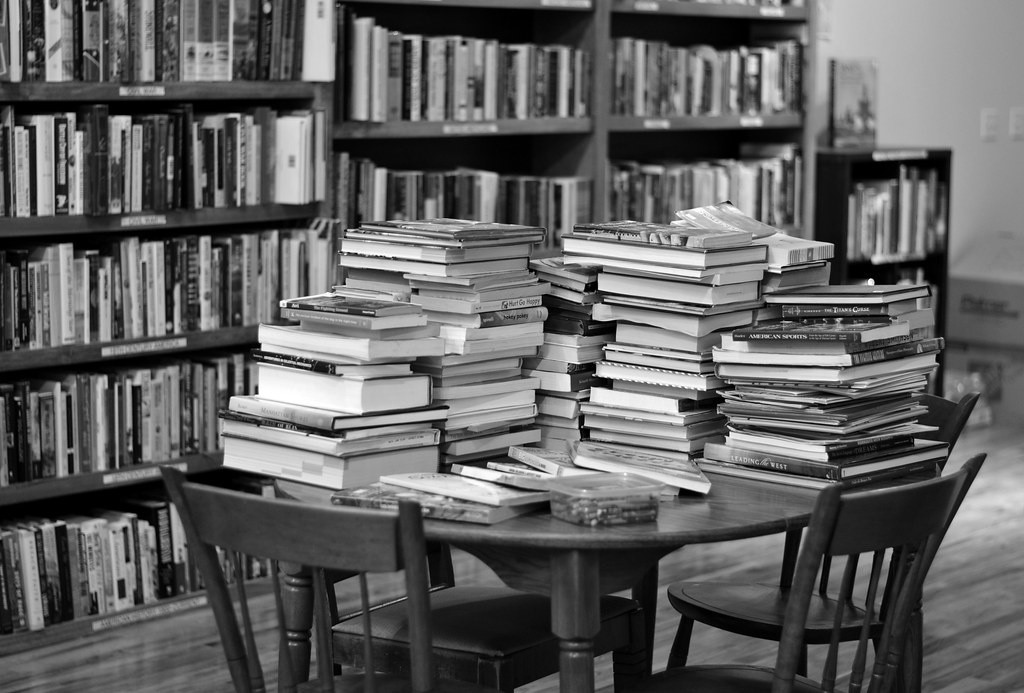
[311,537,649,693]
[665,391,982,693]
[620,453,988,693]
[159,465,506,693]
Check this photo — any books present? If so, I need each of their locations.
[0,104,325,219]
[216,201,951,525]
[634,0,806,5]
[3,228,329,352]
[0,352,259,488]
[844,167,948,262]
[336,151,594,248]
[827,56,879,148]
[610,38,801,116]
[604,143,803,227]
[0,1,336,82]
[334,2,590,122]
[0,476,272,637]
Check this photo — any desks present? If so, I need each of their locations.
[272,472,947,693]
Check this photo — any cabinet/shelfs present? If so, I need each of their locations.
[0,0,819,654]
[818,148,952,398]
